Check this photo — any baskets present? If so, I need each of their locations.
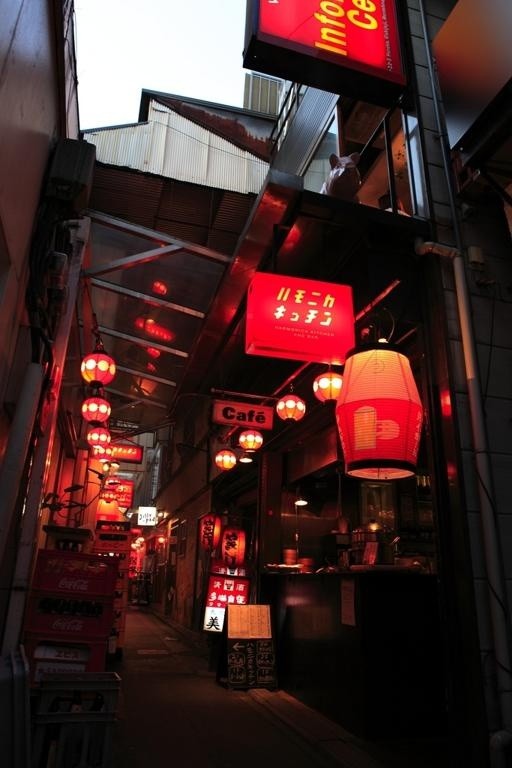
[90,531,131,648]
[22,597,113,688]
[33,549,120,596]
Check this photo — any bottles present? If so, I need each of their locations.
[417,475,431,502]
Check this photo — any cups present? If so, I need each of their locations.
[283,549,298,565]
[299,558,314,574]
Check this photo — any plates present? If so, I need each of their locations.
[278,564,302,570]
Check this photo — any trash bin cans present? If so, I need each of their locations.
[0,671,121,768]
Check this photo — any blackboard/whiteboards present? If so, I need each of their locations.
[228,639,274,688]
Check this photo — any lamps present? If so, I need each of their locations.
[294,485,308,507]
[79,349,124,481]
[196,512,248,569]
[137,264,171,396]
[213,369,344,472]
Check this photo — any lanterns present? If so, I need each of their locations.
[221,523,249,572]
[131,537,145,550]
[213,448,237,472]
[80,344,123,530]
[133,280,175,398]
[239,427,264,453]
[158,537,166,544]
[277,393,310,430]
[200,511,221,557]
[313,372,344,410]
